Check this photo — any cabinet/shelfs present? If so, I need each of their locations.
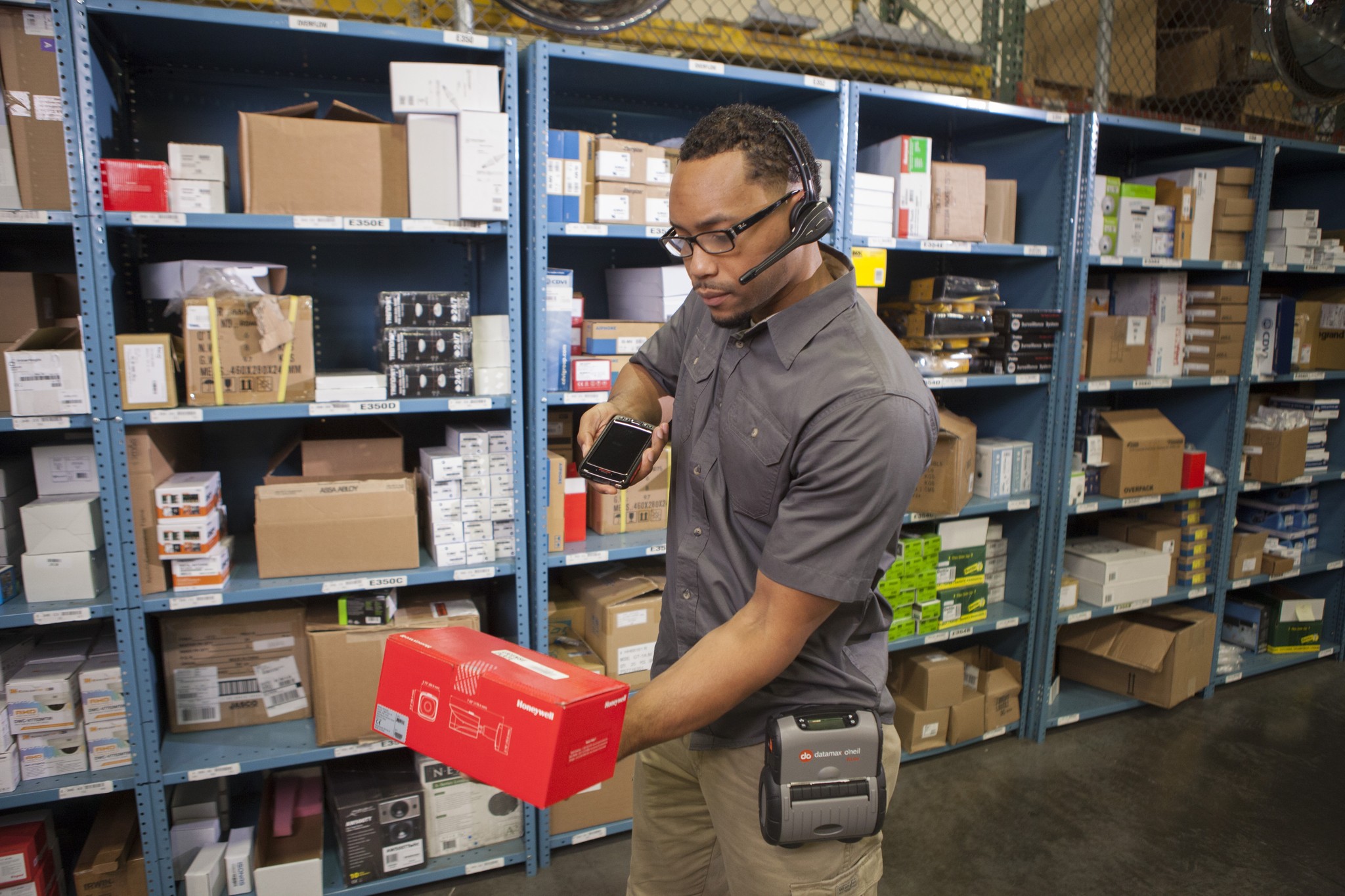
[1213,136,1345,697]
[70,0,549,896]
[0,0,159,896]
[1036,106,1268,747]
[521,34,848,875]
[849,75,1085,755]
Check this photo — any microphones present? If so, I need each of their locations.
[741,202,835,286]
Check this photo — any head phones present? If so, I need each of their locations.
[738,107,819,237]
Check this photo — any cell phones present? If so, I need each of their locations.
[578,414,656,491]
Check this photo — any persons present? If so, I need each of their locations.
[576,102,943,896]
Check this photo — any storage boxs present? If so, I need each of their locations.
[0,13,510,896]
[857,140,1344,736]
[541,116,696,833]
[371,622,628,811]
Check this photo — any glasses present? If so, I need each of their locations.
[657,189,803,256]
